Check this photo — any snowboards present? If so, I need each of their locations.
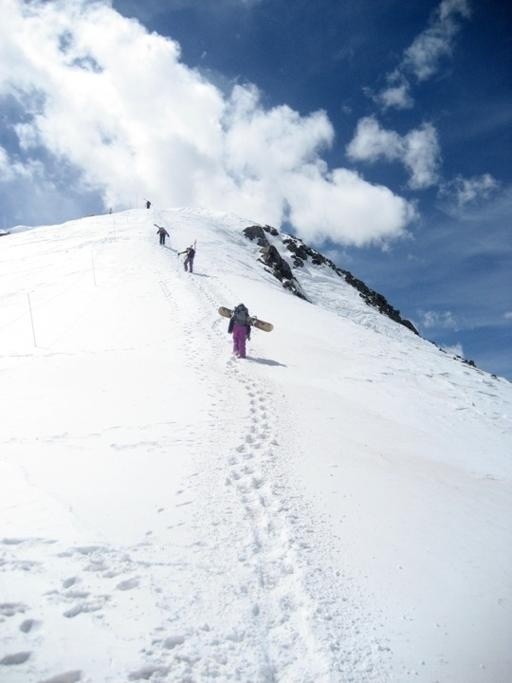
[153,223,169,237]
[218,305,273,332]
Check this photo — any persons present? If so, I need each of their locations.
[146,201,151,209]
[183,245,196,273]
[157,227,169,245]
[227,303,251,358]
